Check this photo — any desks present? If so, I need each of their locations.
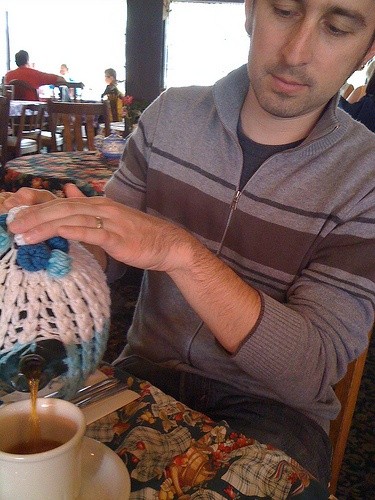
[99,120,137,138]
[2,151,122,197]
[10,100,49,137]
[0,359,336,500]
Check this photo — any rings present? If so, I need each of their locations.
[96,216,102,229]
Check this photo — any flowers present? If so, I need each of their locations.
[120,94,133,118]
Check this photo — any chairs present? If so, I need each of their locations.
[0,79,113,162]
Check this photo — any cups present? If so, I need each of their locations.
[0,397,87,500]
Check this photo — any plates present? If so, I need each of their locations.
[76,436,131,500]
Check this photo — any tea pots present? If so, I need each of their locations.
[93,129,134,161]
[0,209,113,394]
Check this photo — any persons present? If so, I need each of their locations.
[0,0,375,500]
[59,63,72,80]
[4,50,65,130]
[94,68,118,134]
